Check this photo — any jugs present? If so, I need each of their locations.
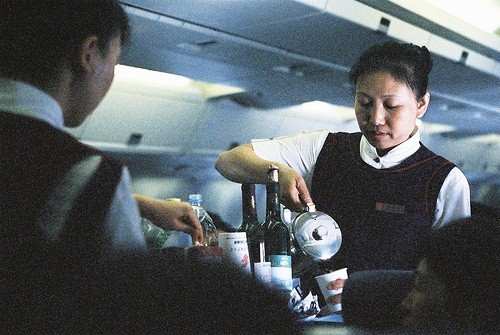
[282,203,342,277]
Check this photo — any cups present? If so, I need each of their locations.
[219,232,251,274]
[315,268,349,313]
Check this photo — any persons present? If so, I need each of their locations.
[190,212,238,255]
[0,0,204,335]
[215,41,471,319]
[64,247,304,335]
[403,217,500,335]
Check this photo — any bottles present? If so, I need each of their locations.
[153,197,193,247]
[236,181,271,287]
[189,193,217,247]
[261,164,292,293]
